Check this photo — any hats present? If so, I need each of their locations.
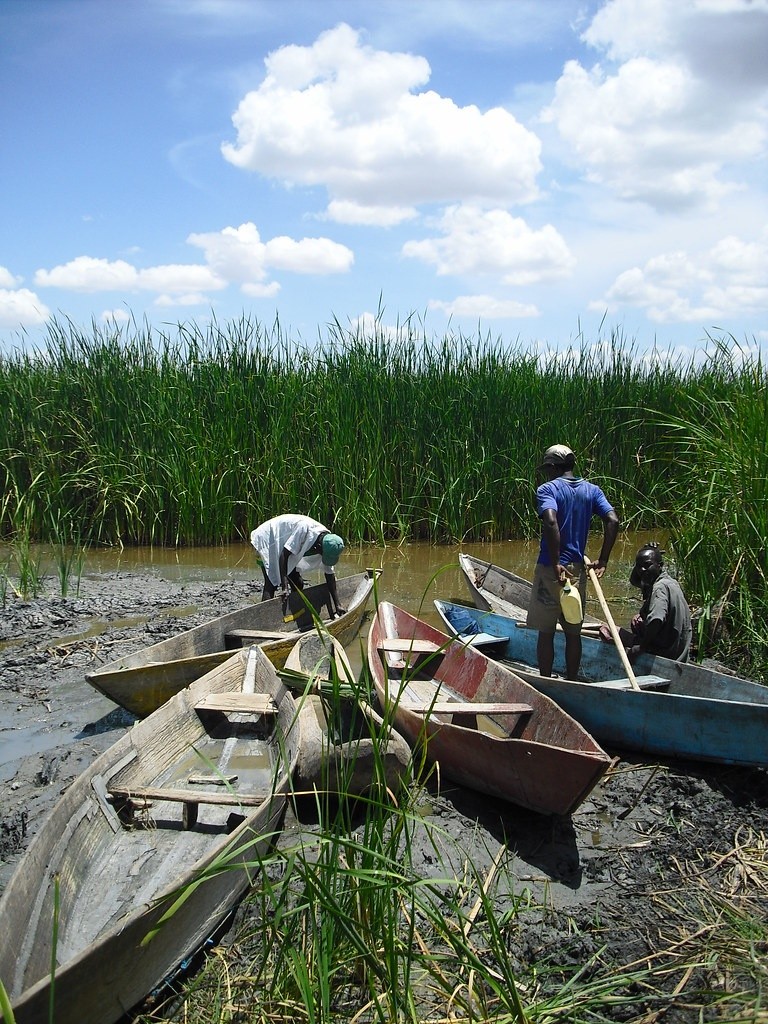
[533,443,577,471]
[322,534,344,566]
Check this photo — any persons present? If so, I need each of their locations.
[525,444,619,682]
[250,514,346,617]
[599,547,692,664]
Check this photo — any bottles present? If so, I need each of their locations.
[560,577,584,625]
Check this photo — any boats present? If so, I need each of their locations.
[0,643,303,1024]
[82,565,384,720]
[367,597,612,820]
[432,597,768,773]
[457,550,632,651]
[280,629,413,802]
